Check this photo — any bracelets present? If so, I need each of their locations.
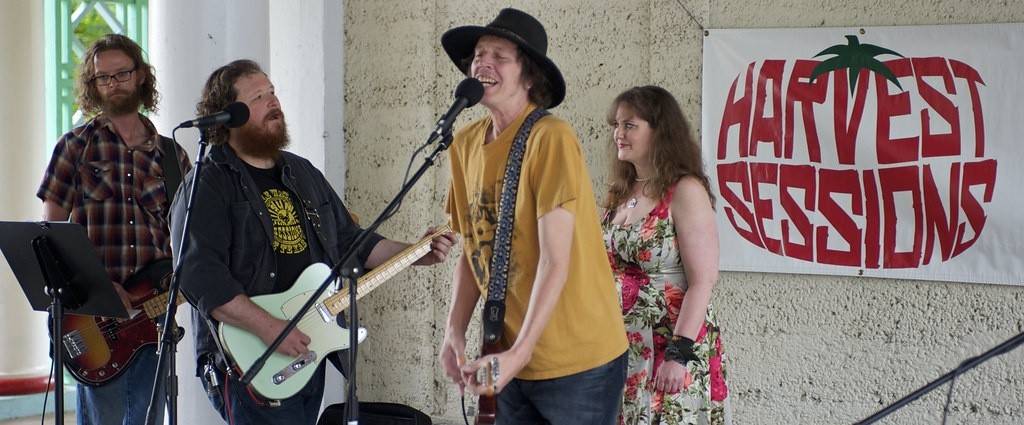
[663,335,702,367]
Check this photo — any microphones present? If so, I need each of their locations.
[428,77,485,144]
[181,101,250,127]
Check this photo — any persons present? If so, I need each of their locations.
[37,33,193,425]
[600,85,733,425]
[440,7,631,425]
[164,59,457,425]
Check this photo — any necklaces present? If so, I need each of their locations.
[626,190,647,208]
[636,176,653,182]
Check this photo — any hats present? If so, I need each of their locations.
[440,7,567,108]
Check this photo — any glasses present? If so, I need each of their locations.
[92,64,140,85]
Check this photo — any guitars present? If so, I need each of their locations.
[46,250,189,387]
[472,316,503,425]
[218,220,453,405]
